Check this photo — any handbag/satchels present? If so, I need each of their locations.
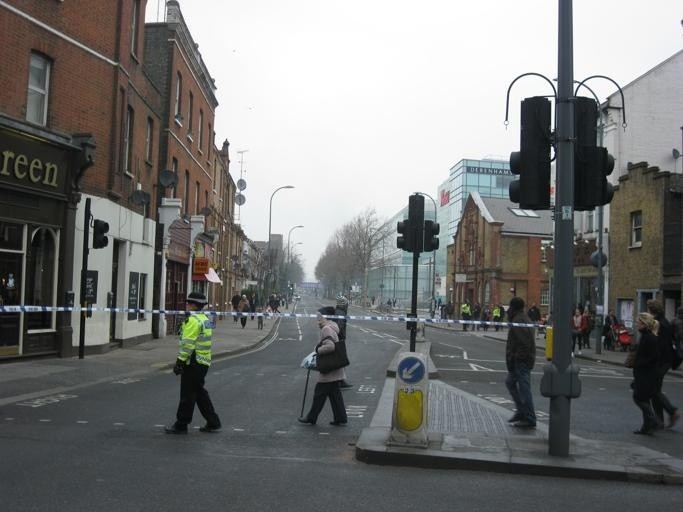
[315,335,351,373]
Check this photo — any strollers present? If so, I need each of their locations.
[608,320,632,352]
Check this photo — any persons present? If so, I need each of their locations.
[330,296,354,389]
[571,308,587,357]
[238,294,250,328]
[581,300,594,350]
[601,308,619,351]
[231,291,242,323]
[582,307,591,349]
[297,306,347,426]
[504,297,536,428]
[471,302,481,331]
[669,304,683,370]
[647,299,680,429]
[164,291,221,435]
[492,304,500,332]
[269,294,281,314]
[482,302,490,332]
[630,313,665,435]
[445,301,453,327]
[248,293,257,320]
[461,299,471,331]
[527,302,541,340]
[498,302,505,331]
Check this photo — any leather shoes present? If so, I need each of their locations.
[331,419,348,425]
[298,417,315,424]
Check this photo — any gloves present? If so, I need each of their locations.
[174,359,185,375]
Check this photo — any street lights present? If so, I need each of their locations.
[268,186,295,295]
[265,185,294,307]
[553,76,610,355]
[368,226,386,302]
[291,242,303,264]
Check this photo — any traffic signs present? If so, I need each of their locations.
[392,352,428,452]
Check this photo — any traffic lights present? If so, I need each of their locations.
[397,219,412,254]
[92,218,109,249]
[396,218,416,253]
[93,217,110,248]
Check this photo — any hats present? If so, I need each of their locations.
[319,307,334,320]
[185,292,209,305]
[336,295,348,309]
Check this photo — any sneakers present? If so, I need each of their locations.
[515,418,536,428]
[635,427,648,435]
[199,420,223,434]
[508,410,525,422]
[666,411,680,430]
[163,421,188,434]
[651,416,663,434]
